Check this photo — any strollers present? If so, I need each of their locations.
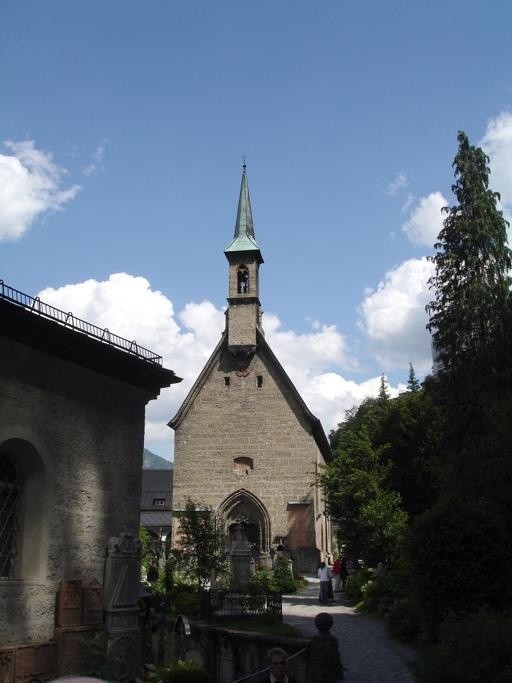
[320,577,336,604]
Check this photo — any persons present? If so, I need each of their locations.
[317,562,330,605]
[147,560,158,586]
[262,647,297,683]
[332,558,361,592]
[305,613,344,683]
